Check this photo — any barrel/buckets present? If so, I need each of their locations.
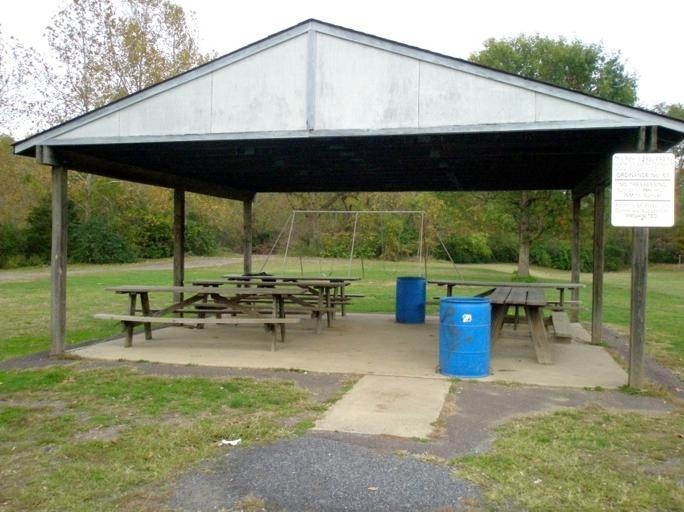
[439,297,492,378]
[396,277,426,323]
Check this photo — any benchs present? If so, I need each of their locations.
[93,313,300,351]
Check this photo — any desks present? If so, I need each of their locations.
[105,274,361,351]
[427,280,585,364]
